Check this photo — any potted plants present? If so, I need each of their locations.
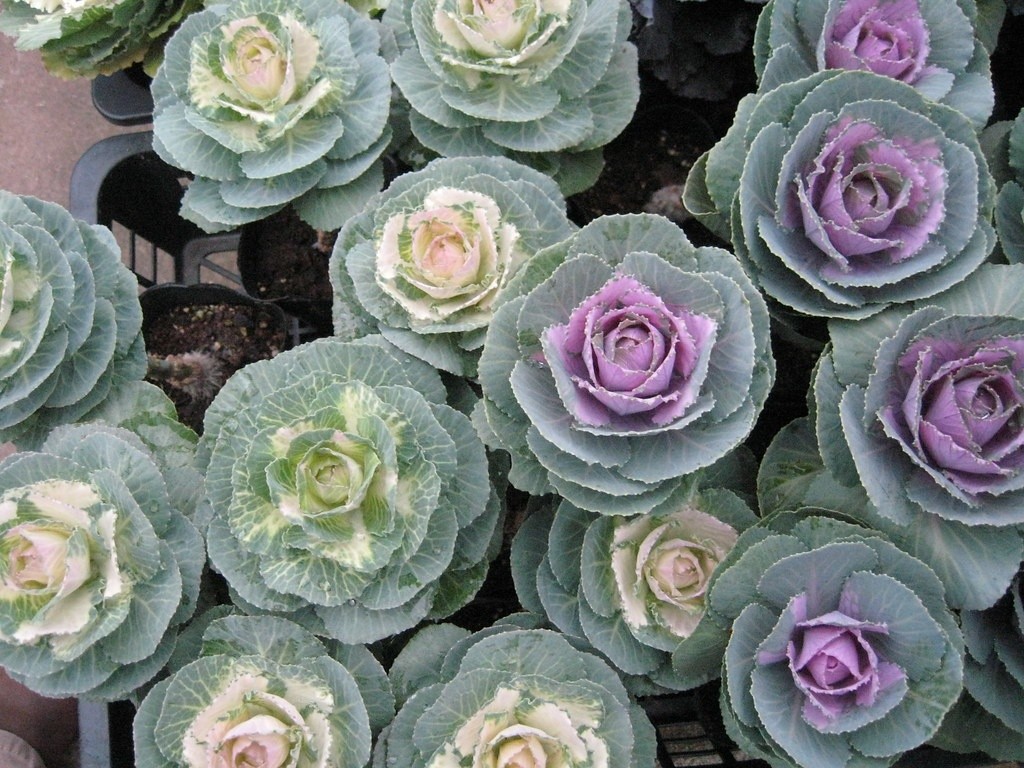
[2,0,1024,768]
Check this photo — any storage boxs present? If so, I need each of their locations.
[67,131,1024,768]
